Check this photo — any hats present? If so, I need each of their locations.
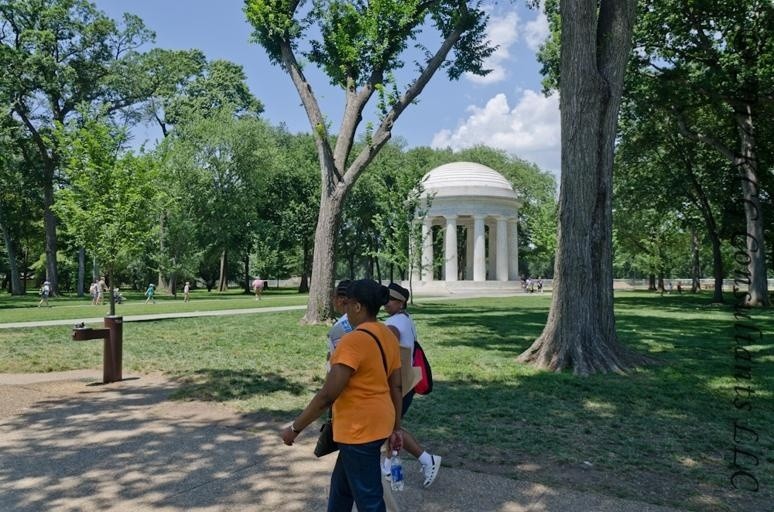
[388,282,410,302]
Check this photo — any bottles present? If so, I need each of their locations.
[390,451,404,491]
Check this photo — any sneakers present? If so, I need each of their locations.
[419,453,442,487]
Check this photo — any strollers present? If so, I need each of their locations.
[112,288,127,304]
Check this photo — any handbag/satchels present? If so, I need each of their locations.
[412,341,433,395]
[314,420,341,458]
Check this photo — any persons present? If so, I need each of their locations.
[182,281,191,303]
[144,283,155,304]
[380,284,441,489]
[677,280,683,294]
[89,276,121,305]
[279,277,405,511]
[37,280,52,308]
[525,276,534,293]
[536,276,543,294]
[322,280,354,384]
[251,275,264,301]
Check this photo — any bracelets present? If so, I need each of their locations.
[290,422,299,434]
